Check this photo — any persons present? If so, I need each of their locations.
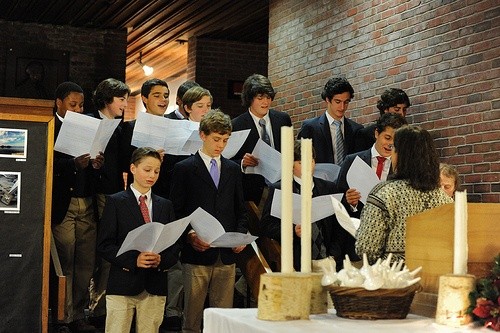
[355,88,411,153]
[51,83,105,333]
[121,78,169,192]
[438,163,462,198]
[77,78,129,319]
[354,125,455,270]
[260,141,336,271]
[166,81,202,119]
[171,110,248,333]
[166,86,214,331]
[297,78,364,184]
[332,113,409,271]
[231,74,292,174]
[97,147,179,333]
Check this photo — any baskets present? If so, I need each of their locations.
[328,283,423,320]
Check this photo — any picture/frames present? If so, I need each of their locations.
[3,47,69,99]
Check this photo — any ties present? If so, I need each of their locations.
[139,195,151,223]
[333,121,345,166]
[260,119,271,148]
[376,156,387,180]
[210,159,219,188]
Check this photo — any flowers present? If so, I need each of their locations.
[467,255,500,332]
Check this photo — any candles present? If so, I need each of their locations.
[281,125,294,273]
[300,137,313,271]
[454,189,467,275]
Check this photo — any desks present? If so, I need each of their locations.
[203,308,494,332]
